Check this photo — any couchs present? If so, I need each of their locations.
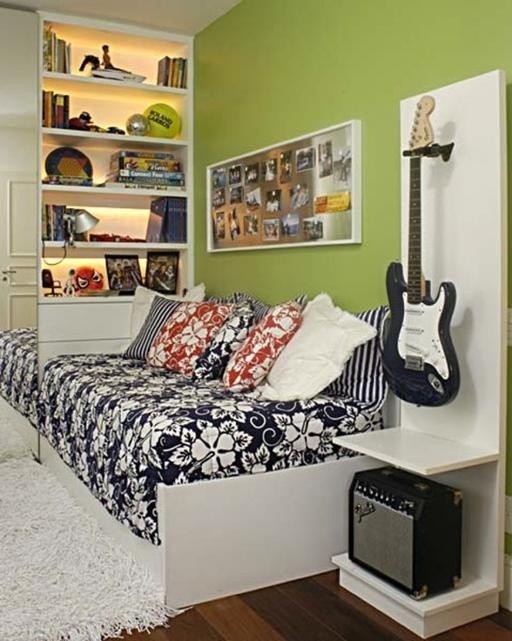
[43,292,400,612]
[0,326,37,456]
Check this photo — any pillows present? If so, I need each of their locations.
[130,281,396,415]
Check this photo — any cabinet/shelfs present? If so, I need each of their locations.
[330,426,507,639]
[38,12,196,386]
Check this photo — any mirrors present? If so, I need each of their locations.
[0,5,38,464]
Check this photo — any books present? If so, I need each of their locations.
[42,26,72,72]
[144,196,186,242]
[42,87,69,129]
[158,56,187,87]
[98,151,186,192]
[42,200,84,241]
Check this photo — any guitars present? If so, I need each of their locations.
[382,96,460,407]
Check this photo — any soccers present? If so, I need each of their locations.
[142,103,183,139]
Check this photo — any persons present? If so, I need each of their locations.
[211,140,352,241]
[107,256,174,290]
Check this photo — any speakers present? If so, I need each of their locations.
[349,467,462,600]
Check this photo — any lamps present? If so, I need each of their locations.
[62,209,100,245]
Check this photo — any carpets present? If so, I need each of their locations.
[0,459,198,640]
